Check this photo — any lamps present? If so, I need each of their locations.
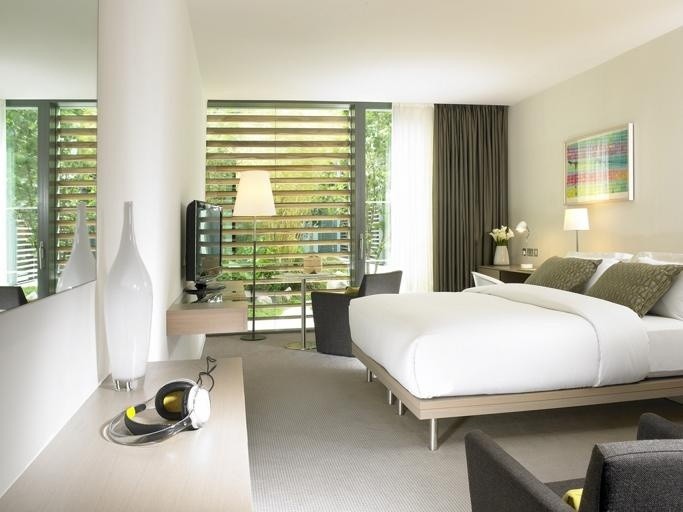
[232,169,277,341]
[563,207,590,251]
[515,221,533,268]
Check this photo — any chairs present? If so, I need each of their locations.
[464,411,683,512]
[310,270,402,357]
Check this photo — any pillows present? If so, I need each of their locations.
[585,260,683,319]
[561,487,583,512]
[522,255,603,293]
[346,286,359,298]
[636,256,683,320]
[564,252,634,296]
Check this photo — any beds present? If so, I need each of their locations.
[348,251,683,451]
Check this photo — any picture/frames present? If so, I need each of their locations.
[563,122,635,206]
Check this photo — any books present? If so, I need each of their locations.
[222,290,246,300]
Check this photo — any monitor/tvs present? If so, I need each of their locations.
[183,200,226,294]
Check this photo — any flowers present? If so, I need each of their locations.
[487,224,515,246]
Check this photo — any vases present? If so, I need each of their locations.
[493,246,510,266]
[56,202,96,294]
[104,200,154,392]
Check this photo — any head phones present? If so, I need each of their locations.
[108,378,211,446]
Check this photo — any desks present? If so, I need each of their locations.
[8,357,253,512]
[479,264,539,283]
[166,281,248,337]
[282,272,337,351]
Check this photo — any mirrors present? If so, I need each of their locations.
[1,98,97,313]
